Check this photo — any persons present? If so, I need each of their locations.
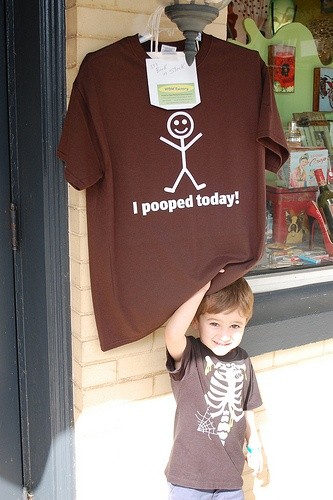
[165,269,271,500]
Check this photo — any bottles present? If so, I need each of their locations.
[314,169,333,243]
